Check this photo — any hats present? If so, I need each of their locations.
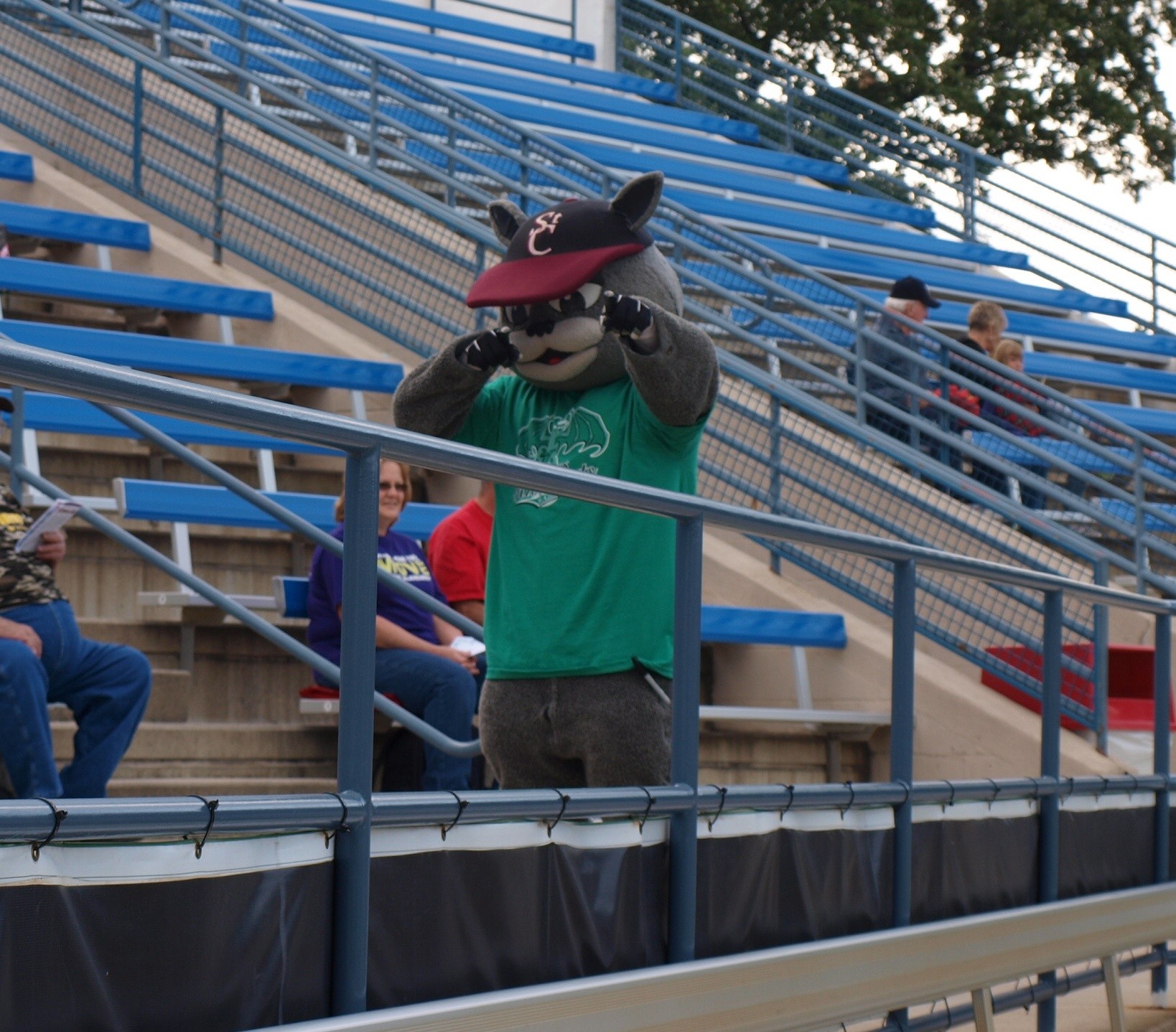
[891,275,942,308]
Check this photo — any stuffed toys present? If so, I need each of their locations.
[394,171,724,789]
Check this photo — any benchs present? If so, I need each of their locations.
[0,0,1176,790]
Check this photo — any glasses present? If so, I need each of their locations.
[380,482,408,491]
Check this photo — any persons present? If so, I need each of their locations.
[424,476,498,627]
[309,456,487,795]
[0,482,154,800]
[843,272,1088,511]
